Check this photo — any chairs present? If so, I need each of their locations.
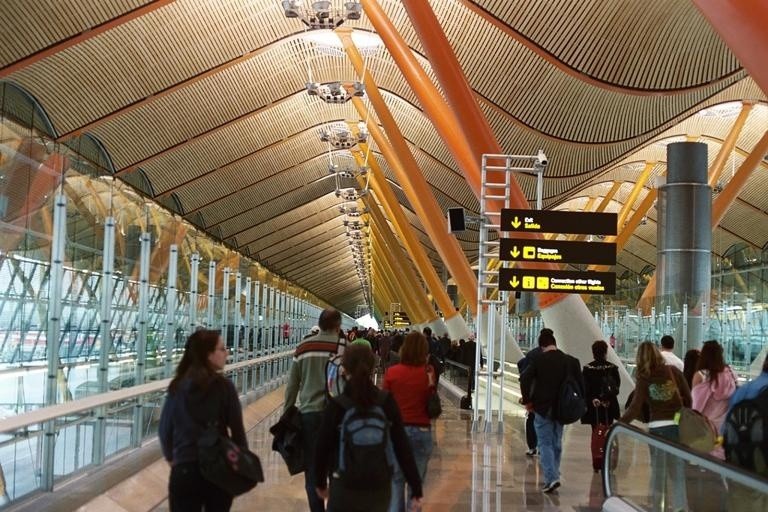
[639,502,660,512]
[666,506,686,512]
[541,480,560,493]
[526,448,536,455]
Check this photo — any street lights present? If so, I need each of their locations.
[724,398,768,479]
[325,353,346,399]
[428,393,441,417]
[197,425,264,496]
[556,370,588,424]
[678,406,716,454]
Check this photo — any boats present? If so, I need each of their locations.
[445,206,466,235]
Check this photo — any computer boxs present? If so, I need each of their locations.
[712,180,723,193]
[538,150,549,167]
[641,216,647,225]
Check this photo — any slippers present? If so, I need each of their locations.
[333,388,394,492]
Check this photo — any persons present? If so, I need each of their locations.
[298,326,480,394]
[158,328,264,510]
[518,325,737,492]
[718,351,768,480]
[378,327,441,512]
[314,338,426,512]
[270,306,351,512]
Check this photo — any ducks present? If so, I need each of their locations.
[591,401,618,473]
